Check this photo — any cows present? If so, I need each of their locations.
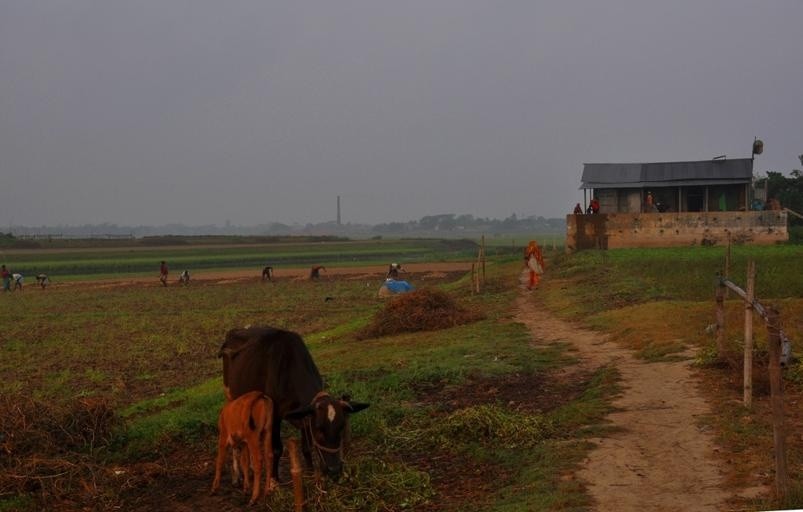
[217,324,373,482]
[207,388,275,508]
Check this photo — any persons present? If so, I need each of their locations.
[527,241,544,291]
[587,197,599,214]
[179,270,190,283]
[574,203,583,214]
[1,265,23,292]
[262,267,273,281]
[310,263,327,279]
[160,259,169,287]
[36,274,48,288]
[647,191,655,210]
[386,262,401,278]
